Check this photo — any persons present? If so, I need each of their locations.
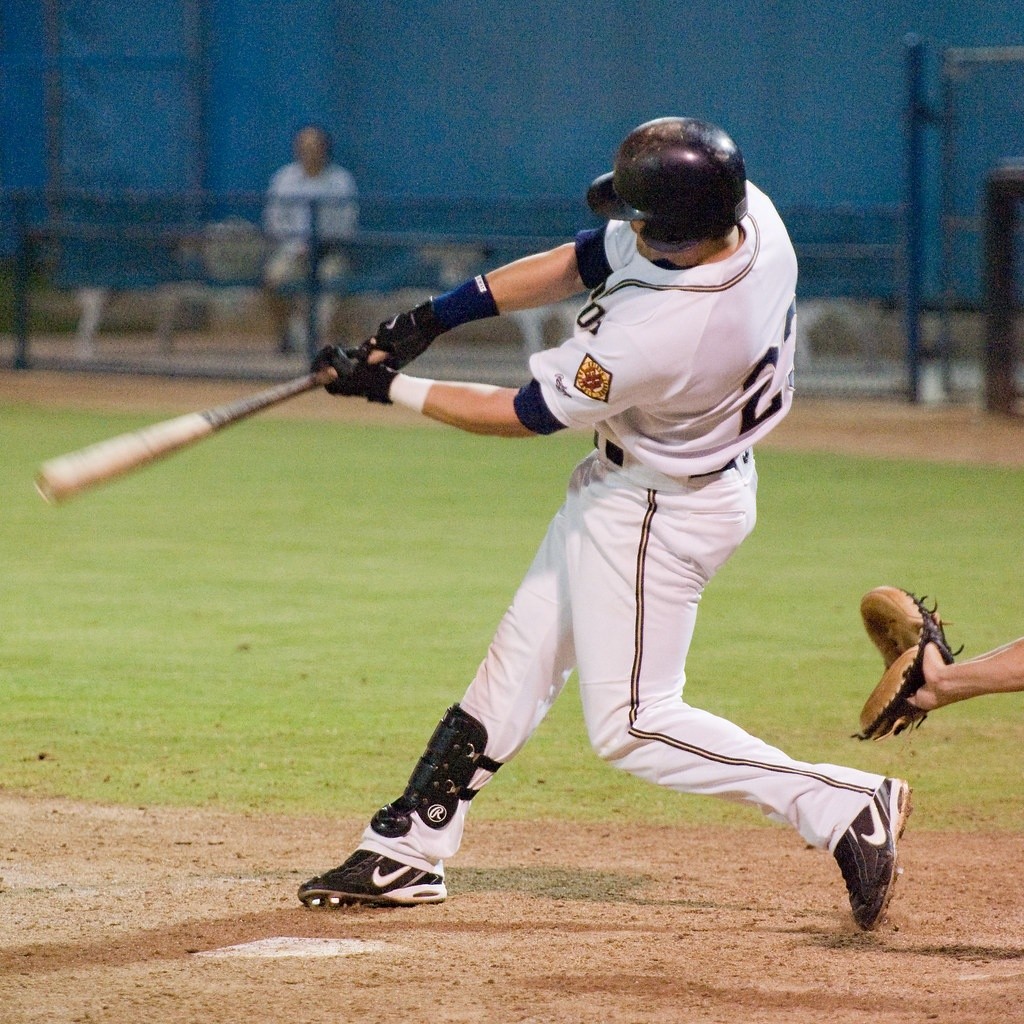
[295,115,915,934]
[904,635,1024,710]
[263,122,362,377]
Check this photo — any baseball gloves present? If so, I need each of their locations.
[853,584,950,740]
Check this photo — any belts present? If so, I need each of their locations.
[592,430,750,480]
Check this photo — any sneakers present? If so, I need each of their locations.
[296,849,448,907]
[832,775,909,932]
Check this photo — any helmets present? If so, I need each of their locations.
[586,116,748,255]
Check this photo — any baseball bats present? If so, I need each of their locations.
[31,349,392,504]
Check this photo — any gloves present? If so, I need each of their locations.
[308,347,396,404]
[343,295,450,374]
